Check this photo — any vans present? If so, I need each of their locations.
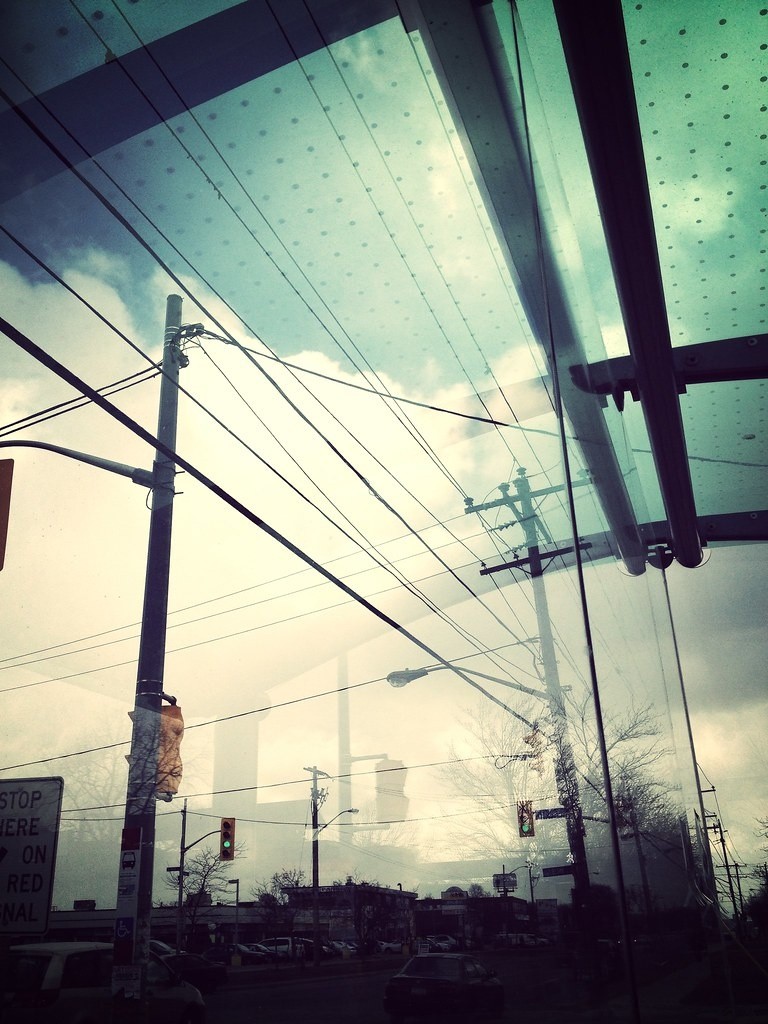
[6,942,208,1024]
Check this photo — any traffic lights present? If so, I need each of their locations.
[218,816,237,863]
[518,800,535,837]
[522,734,545,772]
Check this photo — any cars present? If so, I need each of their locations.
[382,953,506,1024]
[244,943,290,963]
[256,935,480,960]
[206,942,268,966]
[159,953,228,995]
[151,940,174,957]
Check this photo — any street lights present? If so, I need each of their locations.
[227,879,239,957]
[311,807,359,980]
[502,865,529,898]
[397,884,402,891]
[387,631,591,904]
